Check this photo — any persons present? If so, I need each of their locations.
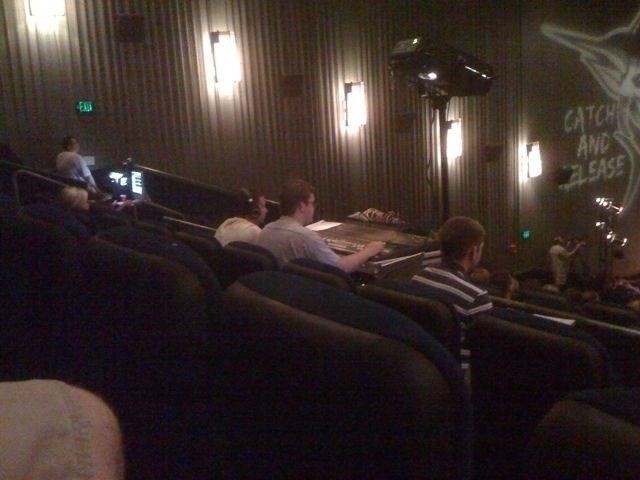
[56,136,106,225]
[539,236,582,277]
[411,216,496,398]
[214,179,386,274]
[0,379,128,480]
[469,266,640,311]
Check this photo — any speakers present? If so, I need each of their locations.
[113,12,145,42]
[558,170,576,186]
[394,111,416,133]
[283,74,306,98]
[482,144,504,164]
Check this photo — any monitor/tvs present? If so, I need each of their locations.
[130,170,143,195]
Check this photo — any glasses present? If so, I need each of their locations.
[305,200,318,208]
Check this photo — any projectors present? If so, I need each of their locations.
[388,34,499,98]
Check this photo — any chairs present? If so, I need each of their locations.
[0,141,640,480]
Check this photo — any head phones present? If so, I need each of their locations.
[240,188,260,218]
[64,143,71,150]
